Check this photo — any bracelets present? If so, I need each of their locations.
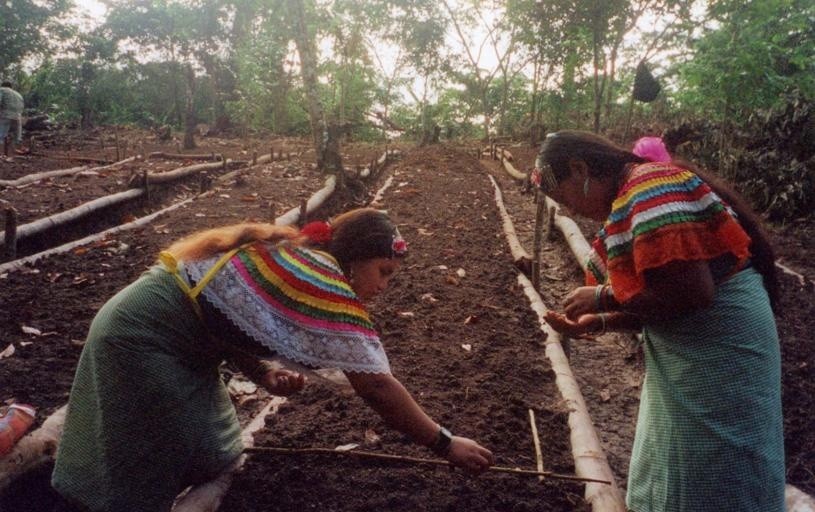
[248,359,273,382]
[585,312,608,337]
[595,282,617,312]
[430,427,453,457]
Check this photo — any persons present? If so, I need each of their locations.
[527,129,790,510]
[47,206,499,512]
[0,81,25,155]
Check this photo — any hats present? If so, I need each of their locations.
[633,136,671,162]
